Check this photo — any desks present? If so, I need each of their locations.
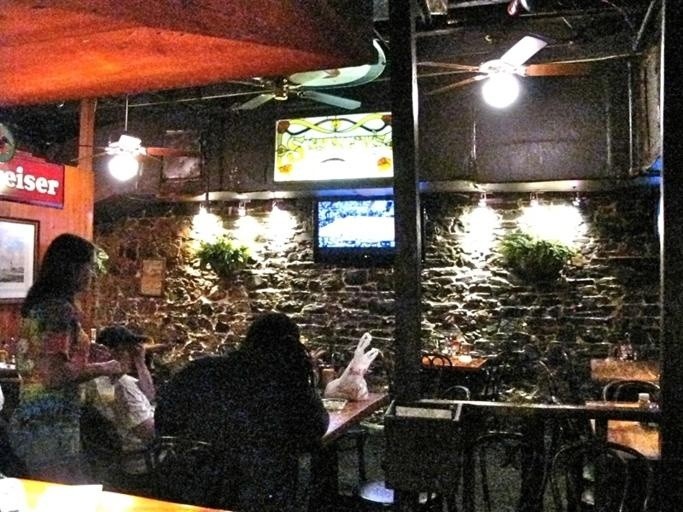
[314,392,391,441]
[586,399,659,459]
[590,358,657,381]
[0,477,227,512]
[423,349,486,372]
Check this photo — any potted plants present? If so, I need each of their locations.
[193,237,255,280]
[493,233,573,288]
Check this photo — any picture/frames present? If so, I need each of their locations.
[0,216,40,306]
[138,256,169,296]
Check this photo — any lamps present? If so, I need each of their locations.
[109,95,144,181]
[481,2,522,108]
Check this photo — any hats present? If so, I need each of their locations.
[91,323,154,351]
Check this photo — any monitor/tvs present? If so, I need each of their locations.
[311,199,394,266]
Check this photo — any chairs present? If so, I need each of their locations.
[550,438,654,510]
[144,435,235,512]
[478,353,506,401]
[601,378,662,404]
[420,352,453,392]
[355,385,471,512]
[331,343,389,478]
[466,432,550,511]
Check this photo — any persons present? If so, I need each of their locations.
[87,326,166,489]
[8,233,122,486]
[153,313,330,512]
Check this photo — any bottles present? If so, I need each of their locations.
[638,393,651,432]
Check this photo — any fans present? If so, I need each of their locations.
[69,132,203,168]
[237,73,362,110]
[415,32,594,98]
